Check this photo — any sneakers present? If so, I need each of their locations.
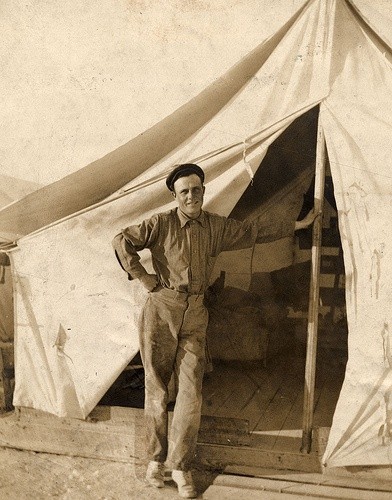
[171,470,198,498]
[146,460,165,488]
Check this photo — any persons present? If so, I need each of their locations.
[111,163,322,498]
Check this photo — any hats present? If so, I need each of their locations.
[166,163,204,191]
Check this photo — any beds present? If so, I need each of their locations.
[130,296,297,366]
